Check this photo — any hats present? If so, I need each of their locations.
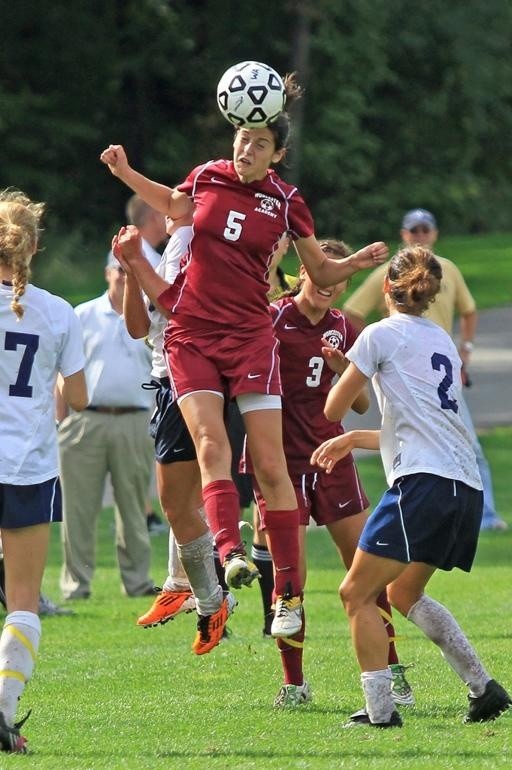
[402,209,436,231]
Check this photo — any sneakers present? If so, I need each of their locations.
[222,540,262,590]
[462,679,512,724]
[271,595,304,637]
[0,709,33,754]
[341,709,404,729]
[388,665,418,706]
[136,588,196,629]
[273,679,313,708]
[39,595,73,618]
[193,590,239,655]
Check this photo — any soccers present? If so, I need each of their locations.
[215,60,287,129]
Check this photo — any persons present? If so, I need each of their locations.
[112,215,238,655]
[101,60,387,639]
[240,239,417,712]
[1,189,87,754]
[311,246,512,731]
[108,195,171,270]
[54,230,162,603]
[344,208,479,371]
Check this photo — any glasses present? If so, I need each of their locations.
[409,226,431,234]
[113,264,123,274]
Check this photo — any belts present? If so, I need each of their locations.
[86,405,149,416]
